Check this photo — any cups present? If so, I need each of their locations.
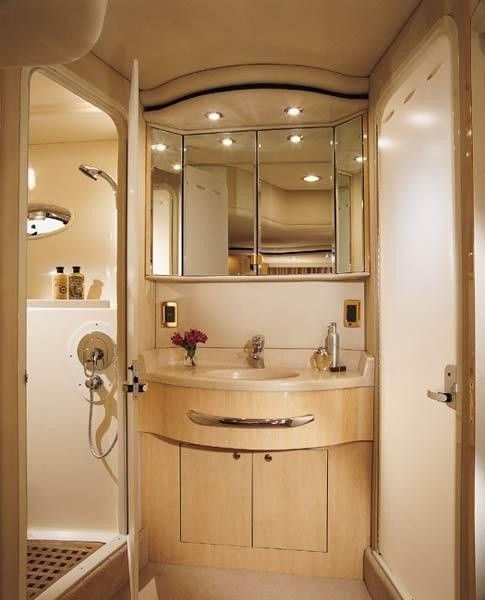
[315,350,332,371]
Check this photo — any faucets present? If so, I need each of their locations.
[249,334,266,369]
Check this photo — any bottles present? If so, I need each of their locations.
[327,322,340,366]
[68,265,85,300]
[52,265,67,300]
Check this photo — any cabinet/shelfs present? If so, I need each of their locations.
[137,380,377,582]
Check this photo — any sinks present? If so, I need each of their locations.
[203,367,300,381]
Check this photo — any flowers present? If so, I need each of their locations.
[170,327,207,366]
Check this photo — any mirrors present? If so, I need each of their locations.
[146,126,183,276]
[183,126,257,277]
[257,126,334,276]
[334,114,365,273]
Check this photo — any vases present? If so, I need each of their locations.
[181,349,200,368]
